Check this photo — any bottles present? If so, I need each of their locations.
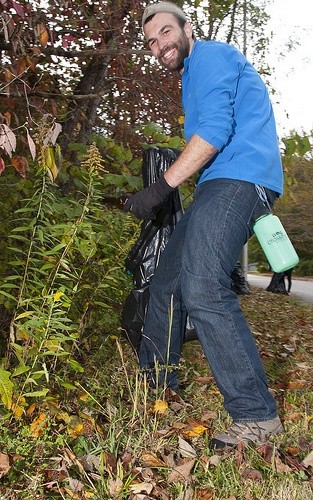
[252,205,299,273]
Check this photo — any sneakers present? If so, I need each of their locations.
[208,414,284,453]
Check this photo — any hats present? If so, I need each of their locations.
[141,2,188,35]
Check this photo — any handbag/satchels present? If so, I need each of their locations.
[120,147,252,350]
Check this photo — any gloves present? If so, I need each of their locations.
[123,176,176,223]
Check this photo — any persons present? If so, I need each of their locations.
[121,2,285,450]
